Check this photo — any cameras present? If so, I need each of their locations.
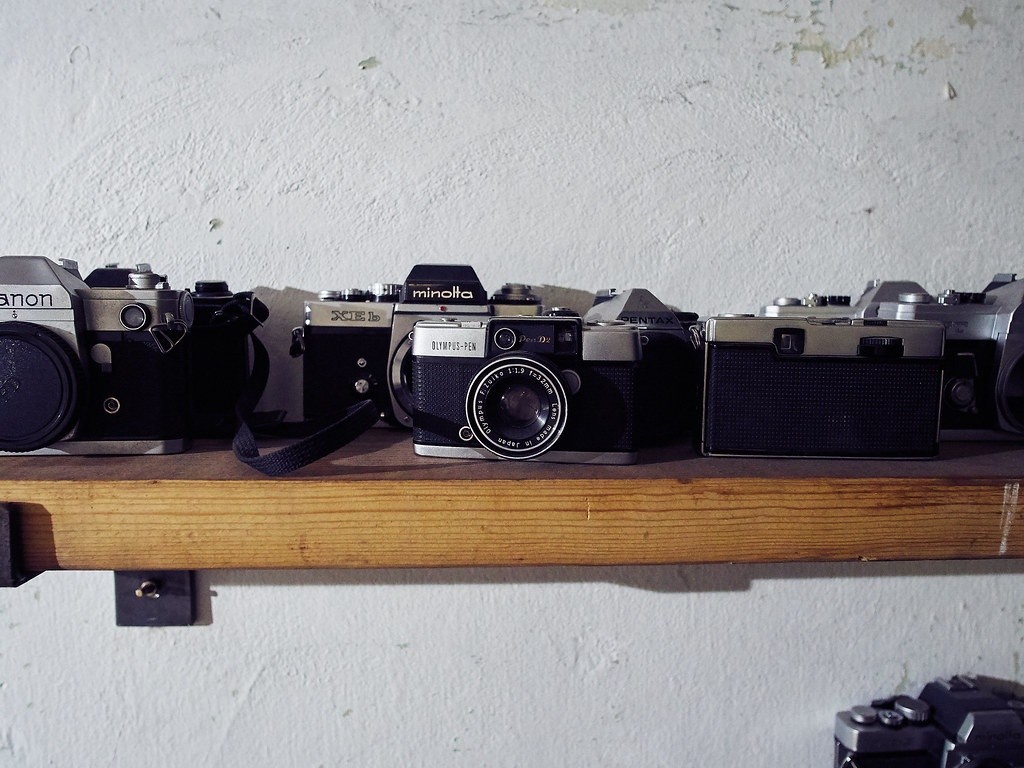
[0,256,234,457]
[834,674,1024,768]
[305,265,1024,466]
